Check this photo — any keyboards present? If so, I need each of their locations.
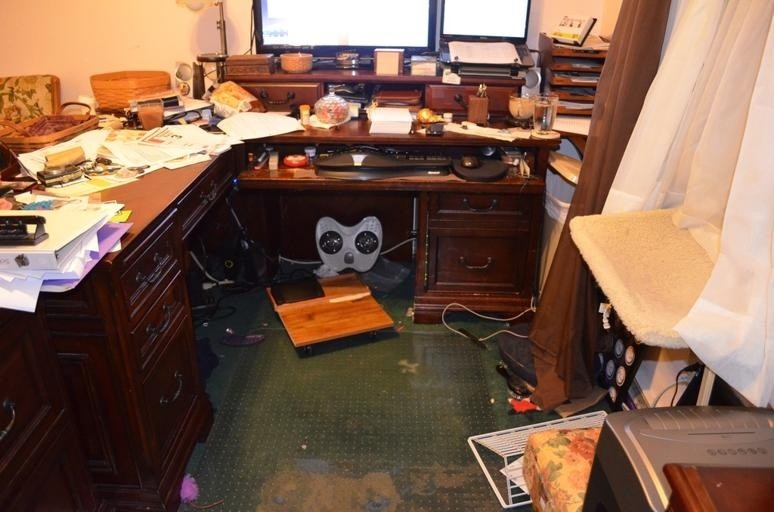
[314,144,450,181]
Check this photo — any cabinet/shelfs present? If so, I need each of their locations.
[220,53,526,127]
[0,111,564,475]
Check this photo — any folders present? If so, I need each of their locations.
[0,208,108,272]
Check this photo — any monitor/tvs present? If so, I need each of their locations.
[253,0,443,71]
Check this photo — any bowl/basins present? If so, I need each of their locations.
[280,53,314,73]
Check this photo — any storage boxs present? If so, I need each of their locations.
[538,33,611,174]
[0,296,53,488]
[468,411,607,508]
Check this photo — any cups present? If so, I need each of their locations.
[509,94,536,121]
[137,98,165,130]
[530,96,559,136]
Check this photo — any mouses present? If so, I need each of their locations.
[461,153,480,167]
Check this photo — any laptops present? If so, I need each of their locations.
[439,0,535,67]
